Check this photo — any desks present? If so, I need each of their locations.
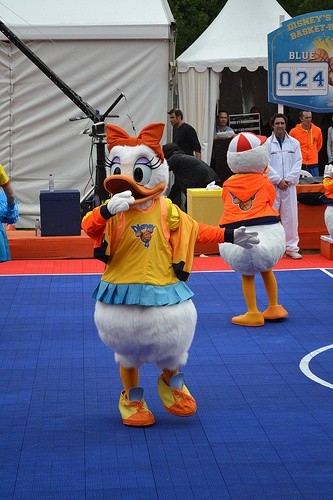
[40,190,81,236]
[187,187,225,255]
[296,182,330,250]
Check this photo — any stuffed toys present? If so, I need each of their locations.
[218,132,289,326]
[81,123,260,426]
[324,161,333,238]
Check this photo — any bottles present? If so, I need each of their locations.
[35,219,41,236]
[49,173,54,193]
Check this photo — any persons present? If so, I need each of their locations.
[156,101,333,238]
[268,114,302,259]
[0,163,18,262]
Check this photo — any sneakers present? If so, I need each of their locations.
[286,251,302,259]
